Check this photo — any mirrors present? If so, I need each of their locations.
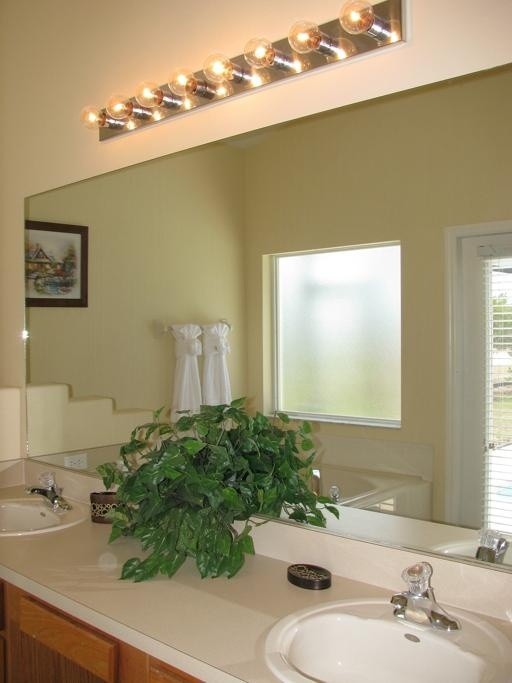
[27,60,511,575]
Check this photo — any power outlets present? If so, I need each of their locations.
[64,453,88,471]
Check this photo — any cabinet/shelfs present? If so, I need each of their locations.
[0,578,202,681]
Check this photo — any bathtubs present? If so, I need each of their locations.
[304,468,378,504]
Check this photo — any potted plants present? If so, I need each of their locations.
[202,399,339,527]
[96,413,264,581]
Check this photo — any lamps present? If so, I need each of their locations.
[77,0,390,127]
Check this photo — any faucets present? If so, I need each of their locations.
[310,468,322,497]
[389,561,461,631]
[24,471,73,511]
[476,530,509,563]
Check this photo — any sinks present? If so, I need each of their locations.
[0,498,60,537]
[265,597,511,683]
[427,540,512,565]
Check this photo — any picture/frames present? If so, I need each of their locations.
[27,219,90,306]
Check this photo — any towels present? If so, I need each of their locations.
[203,322,232,404]
[171,324,202,423]
